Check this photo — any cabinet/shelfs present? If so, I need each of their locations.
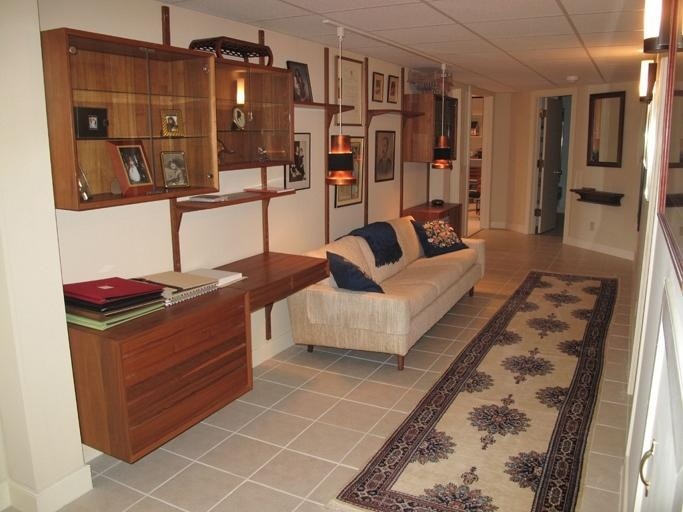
[467,95,482,202]
[165,182,330,340]
[215,59,295,172]
[37,26,219,214]
[401,94,460,163]
[65,283,253,466]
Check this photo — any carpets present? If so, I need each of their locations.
[334,267,624,512]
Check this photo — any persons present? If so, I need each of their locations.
[290,146,304,177]
[378,138,392,176]
[167,117,177,132]
[293,68,305,102]
[127,153,146,183]
[165,159,183,185]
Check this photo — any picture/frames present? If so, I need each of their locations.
[374,130,395,183]
[371,70,384,103]
[332,54,363,127]
[158,108,185,138]
[286,58,313,103]
[158,150,190,188]
[386,74,398,105]
[104,140,155,196]
[332,135,365,208]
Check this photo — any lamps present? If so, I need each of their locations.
[431,60,454,172]
[638,58,656,103]
[641,1,670,55]
[326,24,357,188]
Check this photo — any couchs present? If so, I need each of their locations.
[285,211,487,371]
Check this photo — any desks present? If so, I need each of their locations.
[400,200,463,237]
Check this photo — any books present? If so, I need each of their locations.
[62,266,243,333]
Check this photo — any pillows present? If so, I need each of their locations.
[325,250,384,294]
[409,217,469,257]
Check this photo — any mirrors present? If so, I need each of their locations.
[585,89,625,168]
[657,1,682,288]
